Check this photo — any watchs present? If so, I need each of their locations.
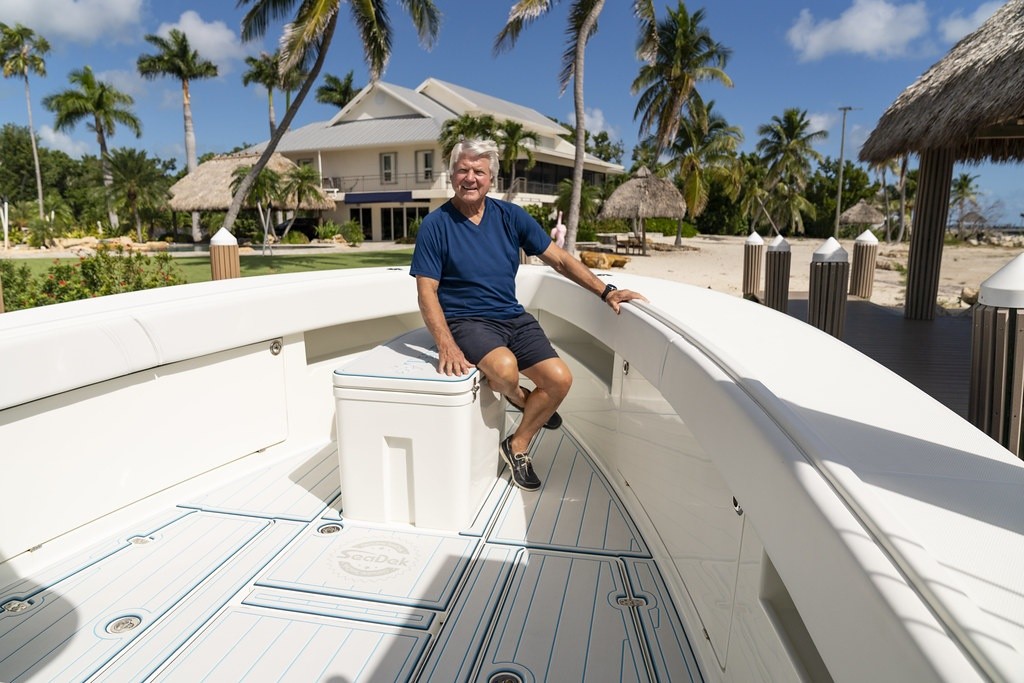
[601,284,617,301]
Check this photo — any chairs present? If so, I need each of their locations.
[615,236,641,255]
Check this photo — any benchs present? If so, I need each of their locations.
[333,323,507,526]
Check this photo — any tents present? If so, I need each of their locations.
[163,153,337,239]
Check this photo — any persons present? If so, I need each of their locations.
[979,229,986,241]
[410,141,649,490]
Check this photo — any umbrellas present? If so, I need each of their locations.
[960,212,986,233]
[839,199,884,233]
[857,0,1024,167]
[599,177,686,254]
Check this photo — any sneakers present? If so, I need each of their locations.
[499,434,542,492]
[504,385,563,430]
